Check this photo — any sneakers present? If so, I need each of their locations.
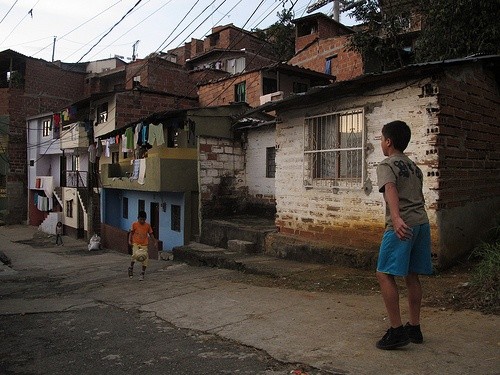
[404,322,423,344]
[376,324,411,350]
[138,272,145,281]
[128,267,133,279]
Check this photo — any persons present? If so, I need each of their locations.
[376,121,432,350]
[128,211,158,281]
[55,221,64,246]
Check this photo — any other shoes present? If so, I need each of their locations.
[56,244,58,246]
[61,244,64,246]
